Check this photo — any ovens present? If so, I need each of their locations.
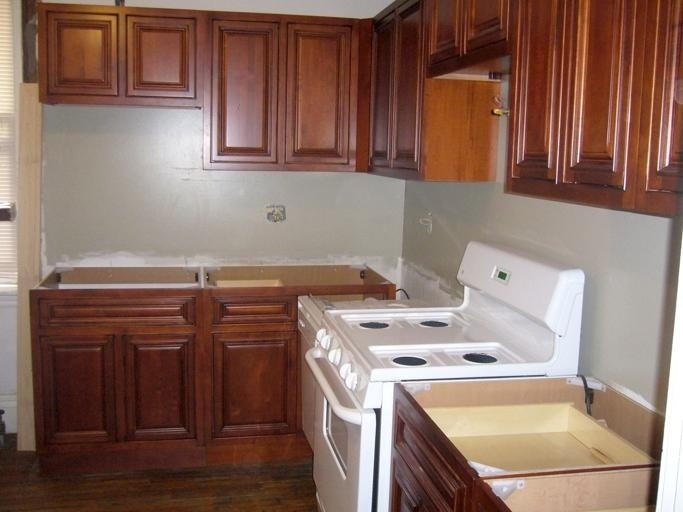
[304,348,394,512]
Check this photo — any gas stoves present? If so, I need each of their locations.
[314,279,556,406]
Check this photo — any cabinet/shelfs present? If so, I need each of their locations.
[389,375,668,512]
